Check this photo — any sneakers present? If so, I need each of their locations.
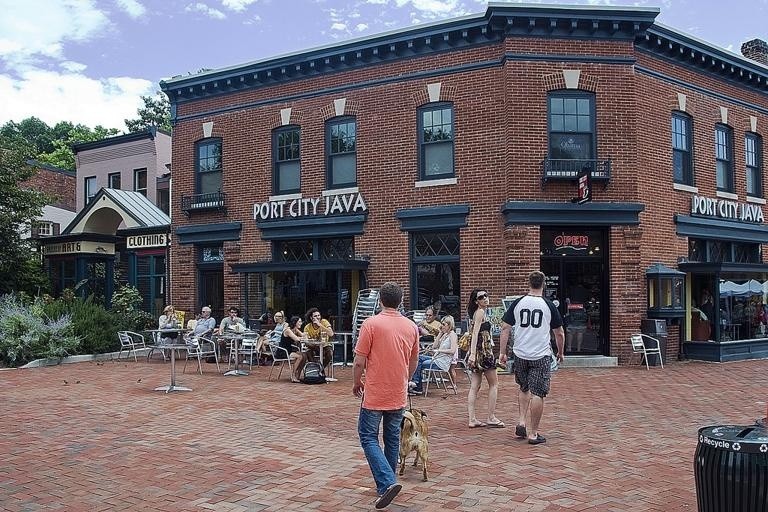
[375,482,403,510]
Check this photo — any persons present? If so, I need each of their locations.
[158,305,335,383]
[352,281,420,509]
[498,270,565,444]
[699,287,768,342]
[565,275,590,352]
[468,287,506,429]
[406,301,459,395]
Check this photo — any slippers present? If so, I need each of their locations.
[488,421,505,428]
[529,433,546,444]
[516,424,526,436]
[469,422,486,428]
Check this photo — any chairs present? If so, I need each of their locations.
[629,332,667,372]
[183,288,477,399]
[117,332,149,363]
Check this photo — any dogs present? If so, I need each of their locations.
[399,408,430,482]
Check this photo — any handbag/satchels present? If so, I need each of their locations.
[459,332,482,351]
[420,335,434,342]
[161,332,178,338]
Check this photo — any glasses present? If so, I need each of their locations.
[476,293,488,300]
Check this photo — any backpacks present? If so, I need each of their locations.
[303,362,326,384]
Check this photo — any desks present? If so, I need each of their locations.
[149,329,191,362]
[721,323,742,341]
[154,344,193,394]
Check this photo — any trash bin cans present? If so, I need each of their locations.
[693,418,768,512]
[639,318,668,366]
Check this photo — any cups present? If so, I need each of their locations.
[320,330,326,344]
[188,323,192,329]
[219,325,224,335]
[235,325,239,333]
[303,332,308,341]
[455,328,461,334]
